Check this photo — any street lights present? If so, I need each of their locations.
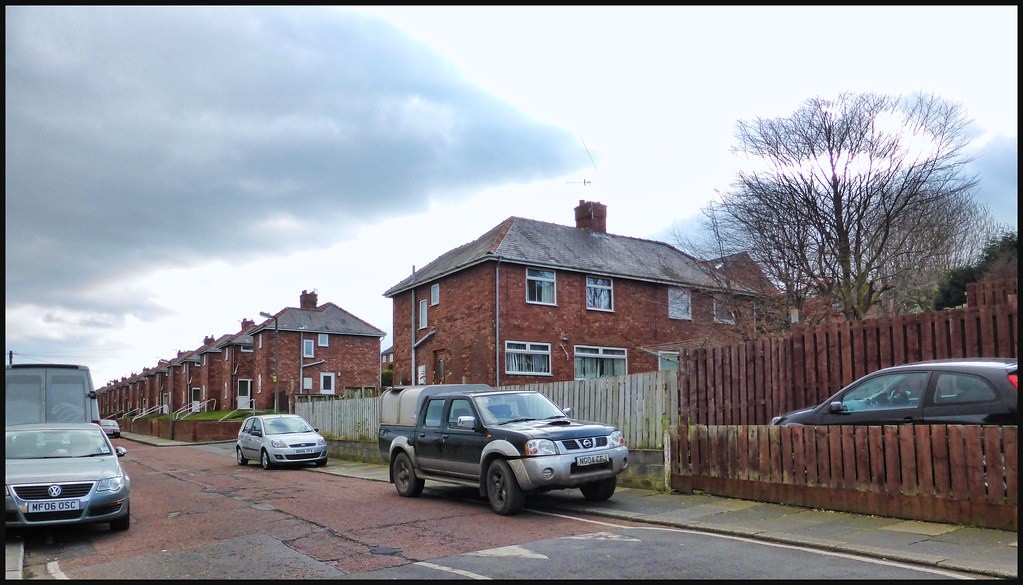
[260,311,279,413]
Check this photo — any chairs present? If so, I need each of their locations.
[917,385,942,405]
[487,404,512,418]
[952,375,979,402]
[70,433,91,454]
[13,435,40,457]
[452,408,470,421]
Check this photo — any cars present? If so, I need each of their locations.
[235,413,329,471]
[5,422,132,532]
[101,419,122,438]
[764,354,1018,491]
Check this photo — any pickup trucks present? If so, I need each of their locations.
[376,384,630,517]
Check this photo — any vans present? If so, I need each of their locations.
[4,362,103,450]
